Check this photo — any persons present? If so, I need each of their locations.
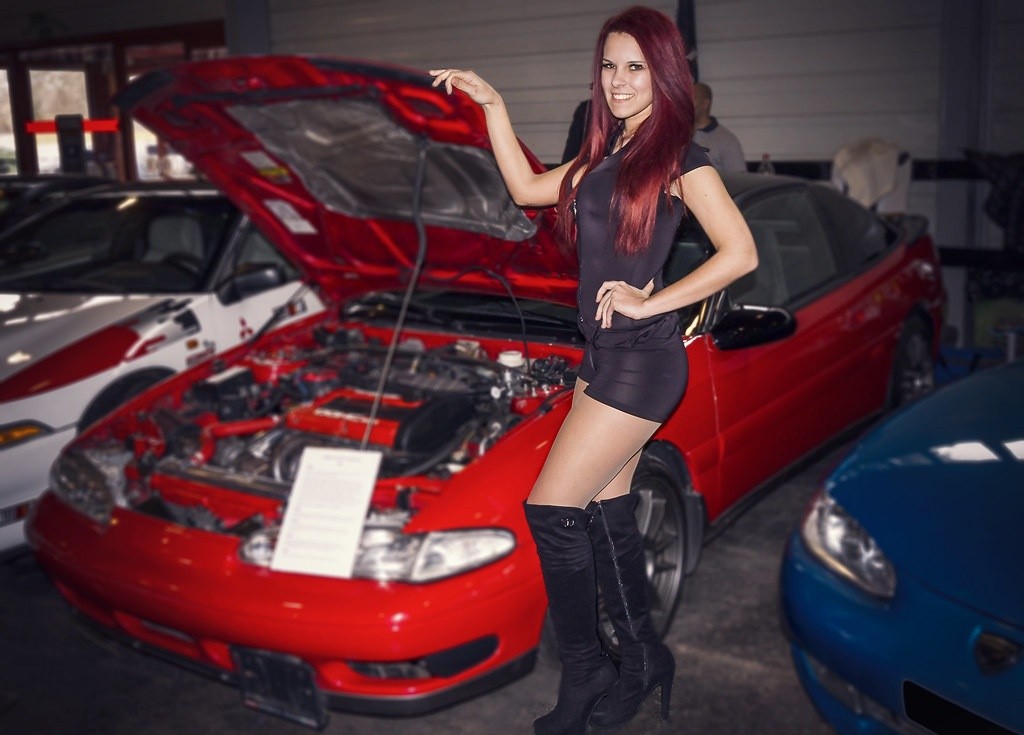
[561,81,594,166]
[428,6,760,735]
[689,82,748,173]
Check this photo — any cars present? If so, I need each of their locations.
[19,54,946,730]
[0,167,327,559]
[771,300,1023,735]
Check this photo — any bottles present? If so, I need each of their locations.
[758,153,774,177]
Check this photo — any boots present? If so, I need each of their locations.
[582,494,679,726]
[523,496,622,734]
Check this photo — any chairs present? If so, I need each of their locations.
[731,220,789,308]
[144,215,206,269]
